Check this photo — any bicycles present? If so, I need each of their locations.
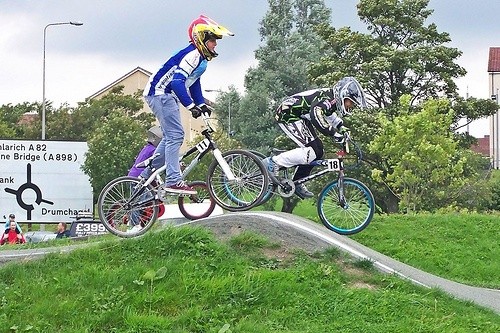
[221,126,376,235]
[97,112,269,238]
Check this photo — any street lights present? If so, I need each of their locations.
[40,20,84,230]
[205,90,231,138]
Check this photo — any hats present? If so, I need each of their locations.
[9,214,15,218]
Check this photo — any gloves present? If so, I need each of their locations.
[334,132,346,147]
[189,105,201,119]
[338,125,351,138]
[200,104,211,117]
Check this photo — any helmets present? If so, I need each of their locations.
[333,76,368,118]
[187,14,235,62]
[147,124,163,146]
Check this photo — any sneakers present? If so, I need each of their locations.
[164,180,198,195]
[138,175,164,192]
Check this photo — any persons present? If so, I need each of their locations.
[0,220,26,246]
[138,13,235,194]
[3,214,23,240]
[261,77,365,197]
[126,125,165,234]
[54,222,70,239]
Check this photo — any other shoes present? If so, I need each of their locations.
[127,224,142,233]
[296,183,314,197]
[261,157,279,186]
